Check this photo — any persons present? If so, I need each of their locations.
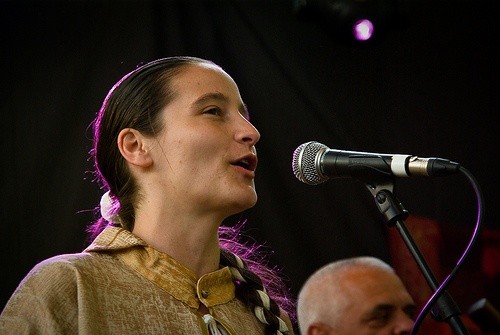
[0,56,299,335]
[297,256,415,335]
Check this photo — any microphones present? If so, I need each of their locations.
[290,141,460,187]
